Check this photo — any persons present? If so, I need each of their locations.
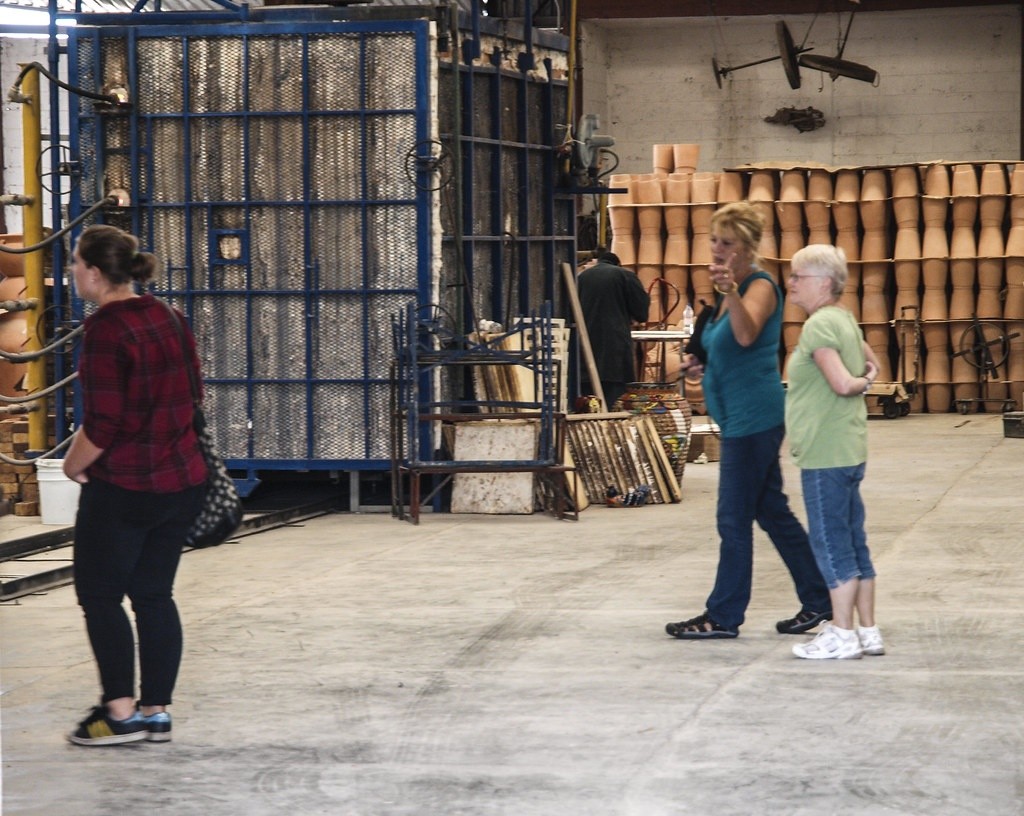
[781,241,885,663]
[570,251,650,413]
[61,225,209,746]
[663,202,832,640]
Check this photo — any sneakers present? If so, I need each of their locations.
[136,700,172,741]
[792,622,863,659]
[858,628,884,655]
[70,705,149,745]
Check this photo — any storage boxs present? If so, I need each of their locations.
[687,431,721,462]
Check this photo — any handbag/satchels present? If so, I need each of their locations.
[183,408,244,548]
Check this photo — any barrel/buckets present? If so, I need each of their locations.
[35,457,83,526]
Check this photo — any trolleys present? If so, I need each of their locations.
[864,305,921,418]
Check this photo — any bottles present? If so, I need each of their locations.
[683,303,694,334]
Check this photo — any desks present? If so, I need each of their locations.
[632,329,694,396]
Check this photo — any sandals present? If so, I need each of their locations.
[666,612,739,638]
[776,610,832,634]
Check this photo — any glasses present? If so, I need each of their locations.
[790,274,817,282]
